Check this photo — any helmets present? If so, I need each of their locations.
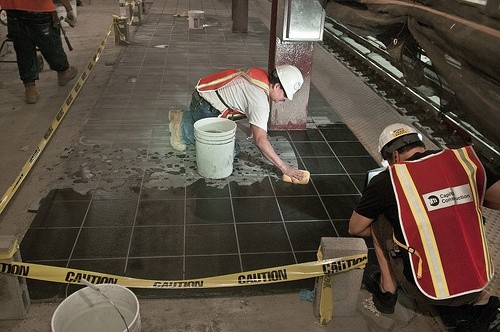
[377,122,418,153]
[275,64,304,101]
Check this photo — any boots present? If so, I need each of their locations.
[58,66,78,87]
[25,86,40,104]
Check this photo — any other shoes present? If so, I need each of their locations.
[168,110,183,121]
[366,272,399,314]
[169,120,186,152]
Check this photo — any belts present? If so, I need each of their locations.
[193,92,202,104]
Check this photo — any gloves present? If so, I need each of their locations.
[0,10,7,24]
[67,9,76,27]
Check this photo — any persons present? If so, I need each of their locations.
[349,123,500,313]
[0,0,78,104]
[169,65,305,184]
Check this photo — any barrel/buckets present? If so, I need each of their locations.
[192,117,237,180]
[188,10,205,29]
[50,277,142,332]
